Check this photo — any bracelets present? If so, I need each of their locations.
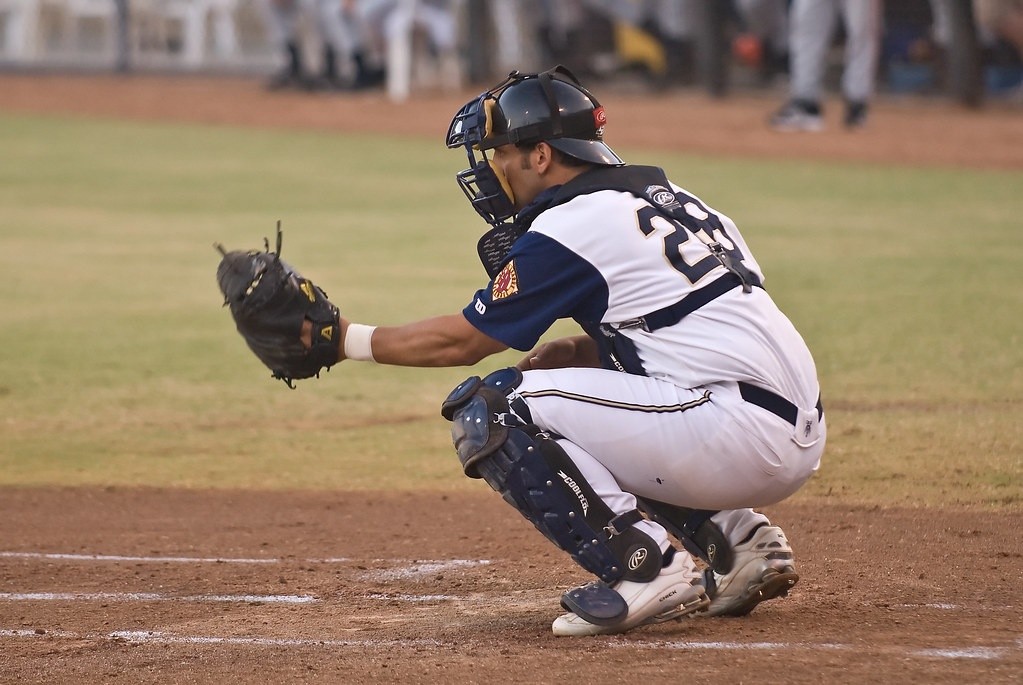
[344,322,378,362]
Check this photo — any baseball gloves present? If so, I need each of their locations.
[215,245,340,382]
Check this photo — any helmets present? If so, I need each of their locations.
[490,78,625,166]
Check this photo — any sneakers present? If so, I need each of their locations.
[689,524,799,618]
[552,550,708,638]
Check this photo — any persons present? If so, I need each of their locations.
[268,0,396,91]
[766,0,882,134]
[216,64,828,636]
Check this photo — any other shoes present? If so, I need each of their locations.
[843,102,867,129]
[762,102,824,132]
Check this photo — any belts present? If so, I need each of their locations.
[738,381,822,426]
